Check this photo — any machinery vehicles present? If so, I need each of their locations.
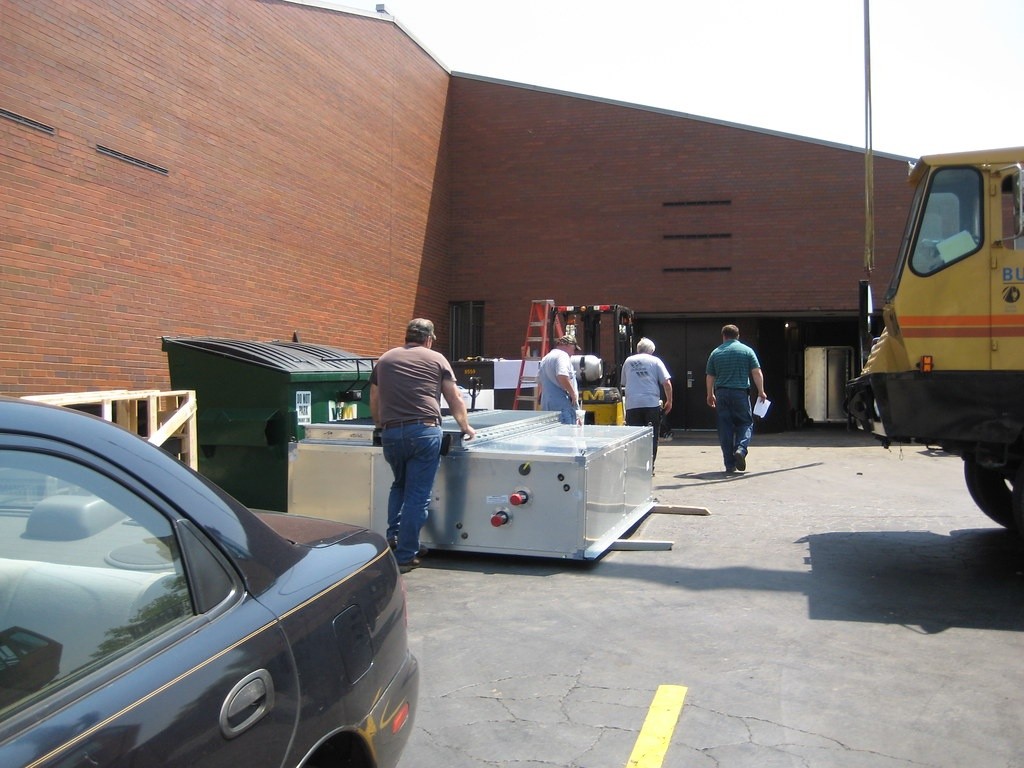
[533,302,637,427]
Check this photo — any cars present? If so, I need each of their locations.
[1,393,419,767]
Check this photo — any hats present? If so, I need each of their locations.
[406,317,437,340]
[559,335,582,350]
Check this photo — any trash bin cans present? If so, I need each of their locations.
[158,328,377,514]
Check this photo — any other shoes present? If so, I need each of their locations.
[726,466,736,473]
[735,449,746,471]
[390,535,398,548]
[399,558,421,572]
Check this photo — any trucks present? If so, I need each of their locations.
[841,145,1023,538]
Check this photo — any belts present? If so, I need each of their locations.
[718,386,749,391]
[386,418,436,428]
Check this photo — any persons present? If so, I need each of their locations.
[537,334,581,424]
[706,324,767,473]
[369,317,475,569]
[621,337,672,477]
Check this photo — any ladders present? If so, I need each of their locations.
[512,299,564,410]
